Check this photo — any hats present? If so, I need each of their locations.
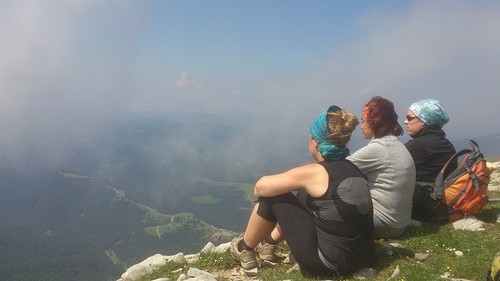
[410,98,449,130]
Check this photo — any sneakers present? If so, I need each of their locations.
[254,241,275,261]
[230,233,257,269]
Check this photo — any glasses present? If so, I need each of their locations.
[406,114,416,120]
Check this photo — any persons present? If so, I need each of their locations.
[403,98,460,222]
[230,106,373,275]
[344,95,415,240]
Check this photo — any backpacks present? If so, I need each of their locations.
[433,138,496,225]
